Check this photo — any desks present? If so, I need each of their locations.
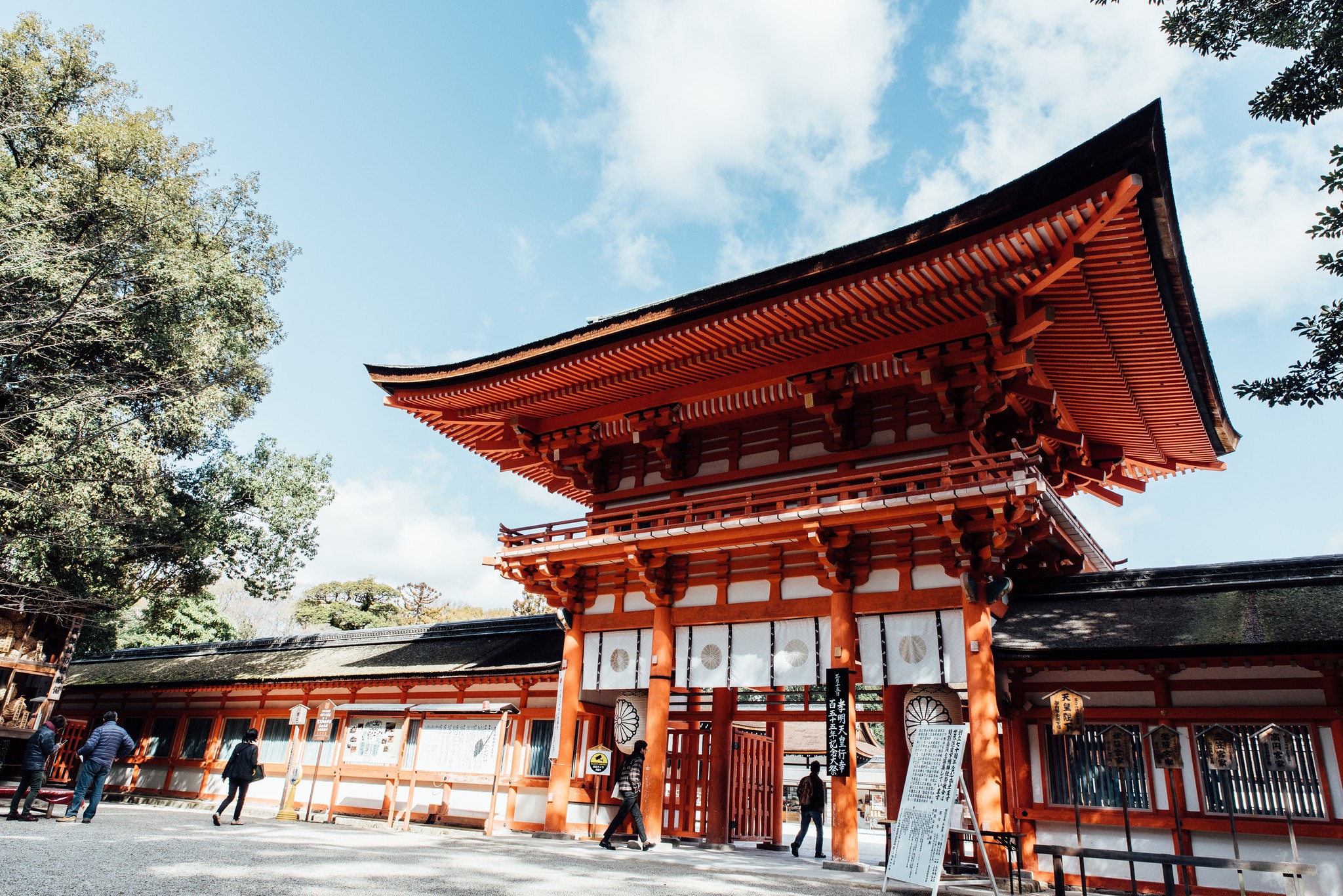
[876,819,1027,896]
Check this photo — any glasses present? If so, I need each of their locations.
[644,748,648,752]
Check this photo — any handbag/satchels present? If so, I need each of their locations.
[611,781,624,800]
[248,764,266,784]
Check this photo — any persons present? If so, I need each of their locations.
[212,727,258,826]
[857,798,864,811]
[5,714,68,822]
[790,760,826,858]
[598,740,656,851]
[55,711,134,824]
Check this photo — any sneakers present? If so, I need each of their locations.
[791,842,800,857]
[18,812,39,821]
[6,811,20,821]
[82,819,91,823]
[815,852,826,858]
[213,812,221,826]
[56,816,76,822]
[231,818,244,825]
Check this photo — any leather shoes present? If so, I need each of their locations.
[642,842,656,851]
[599,839,616,850]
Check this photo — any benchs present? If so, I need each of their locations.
[0,786,85,820]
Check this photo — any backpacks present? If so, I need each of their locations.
[799,777,820,814]
